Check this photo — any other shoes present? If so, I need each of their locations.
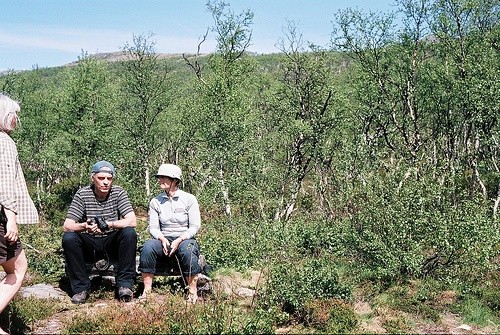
[71,290,89,304]
[119,286,133,298]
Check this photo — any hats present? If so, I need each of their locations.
[91,160,115,175]
[157,164,183,181]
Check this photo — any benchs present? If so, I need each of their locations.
[85,254,205,277]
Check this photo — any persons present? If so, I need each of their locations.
[0,95,39,335]
[139,164,202,302]
[62,161,137,302]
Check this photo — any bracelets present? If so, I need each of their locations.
[112,221,114,229]
[179,237,183,241]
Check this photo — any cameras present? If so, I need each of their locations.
[94,216,109,232]
